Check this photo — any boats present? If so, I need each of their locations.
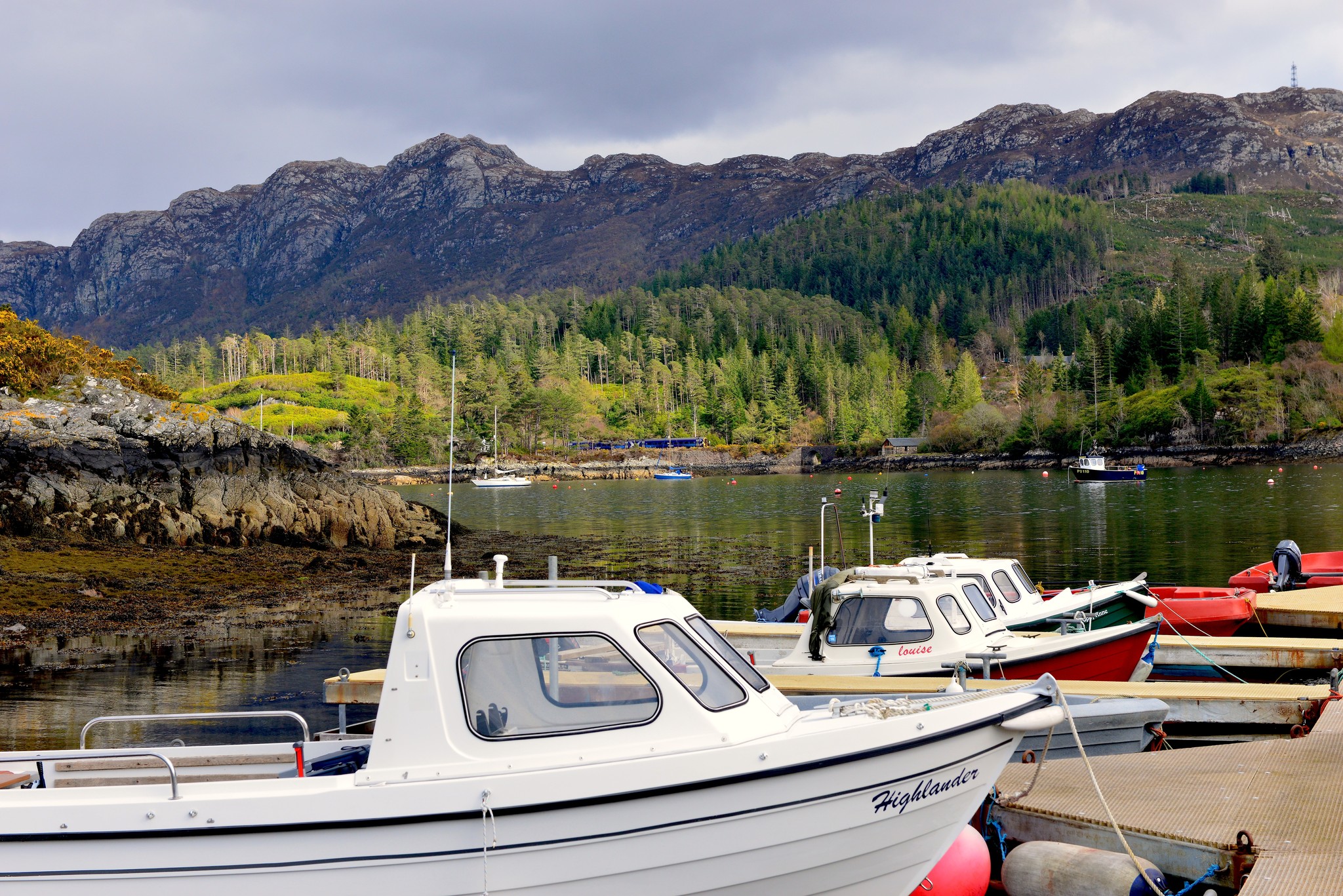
[1068,429,1148,482]
[1229,539,1343,590]
[743,495,1163,681]
[1041,571,1260,636]
[844,493,1159,632]
[0,351,1056,896]
[301,656,1167,763]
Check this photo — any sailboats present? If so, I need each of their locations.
[654,423,693,480]
[471,405,532,487]
